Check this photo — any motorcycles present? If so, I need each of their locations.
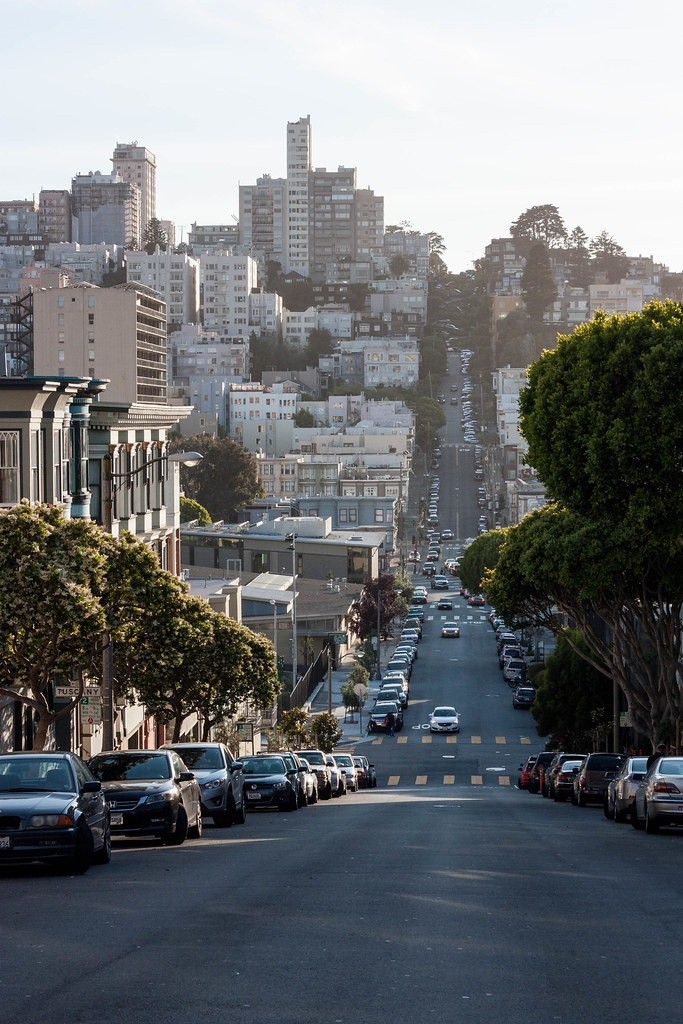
[508,673,534,688]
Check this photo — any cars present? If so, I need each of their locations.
[410,585,428,604]
[629,755,683,834]
[428,706,462,733]
[422,345,489,576]
[408,551,420,562]
[437,599,453,610]
[459,583,485,606]
[488,606,523,670]
[516,751,589,802]
[236,748,378,813]
[84,748,203,845]
[0,749,113,875]
[430,574,449,590]
[602,756,652,824]
[440,621,461,638]
[367,607,425,734]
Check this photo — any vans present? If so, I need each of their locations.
[502,657,526,682]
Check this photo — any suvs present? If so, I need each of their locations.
[157,741,246,828]
[511,686,536,708]
[571,751,630,807]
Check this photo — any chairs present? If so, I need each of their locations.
[47,769,71,787]
[0,775,20,790]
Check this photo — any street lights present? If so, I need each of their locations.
[99,450,206,750]
[327,651,366,754]
[413,524,424,575]
[376,588,403,676]
